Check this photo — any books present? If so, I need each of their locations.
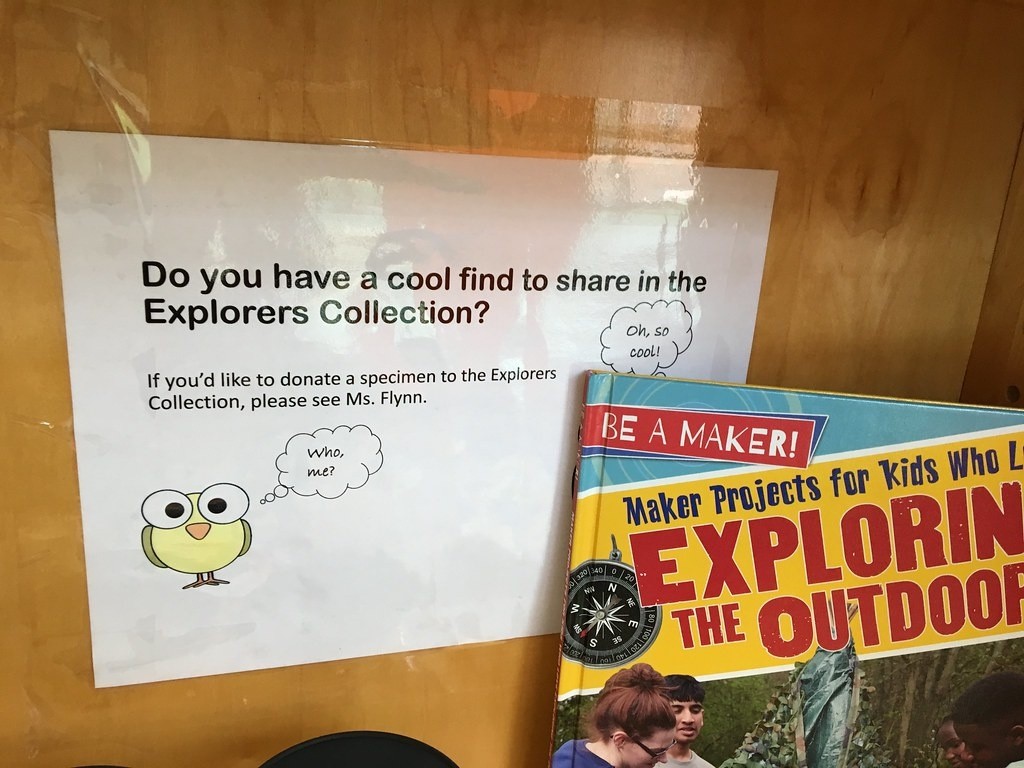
[549,371,1024,768]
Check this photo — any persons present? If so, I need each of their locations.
[935,712,973,765]
[655,674,714,768]
[953,671,1024,768]
[554,662,676,768]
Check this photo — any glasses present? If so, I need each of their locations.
[609,732,677,758]
